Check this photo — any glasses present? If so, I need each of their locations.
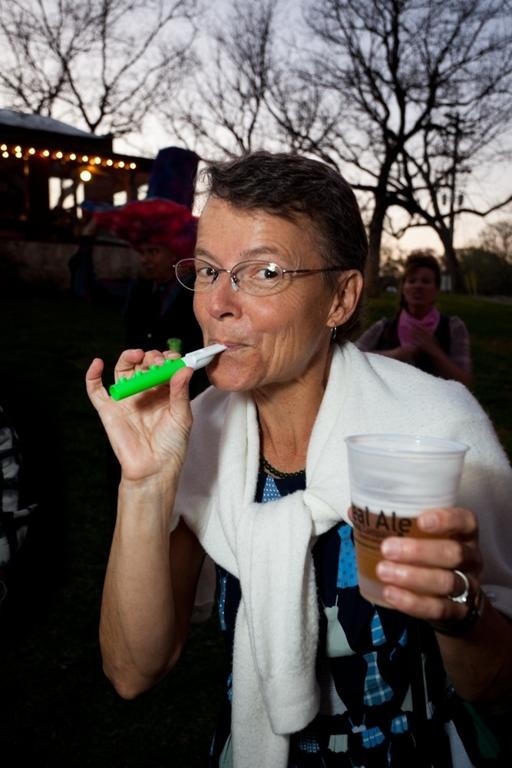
[172,257,352,296]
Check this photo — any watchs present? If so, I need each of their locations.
[430,588,485,637]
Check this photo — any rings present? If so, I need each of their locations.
[446,569,471,604]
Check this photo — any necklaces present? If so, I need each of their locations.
[261,455,305,479]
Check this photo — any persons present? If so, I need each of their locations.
[69,196,210,400]
[354,252,474,390]
[85,153,512,768]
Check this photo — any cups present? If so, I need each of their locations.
[345,435,470,610]
